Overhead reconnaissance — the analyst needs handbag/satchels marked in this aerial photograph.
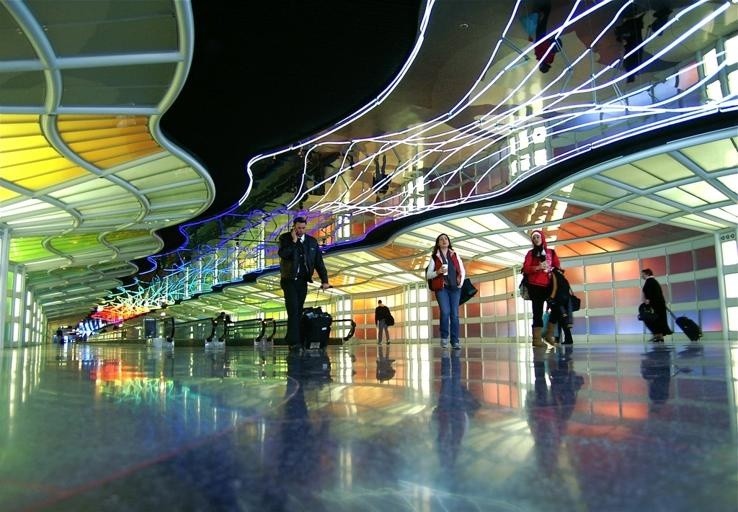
[459,278,478,305]
[570,295,580,312]
[519,273,532,300]
[385,314,394,325]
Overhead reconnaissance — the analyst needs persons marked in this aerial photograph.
[270,351,334,486]
[523,230,561,347]
[432,355,481,487]
[213,311,232,323]
[637,346,672,396]
[613,10,645,83]
[374,300,392,344]
[364,344,397,391]
[551,346,589,447]
[426,234,465,348]
[56,326,63,345]
[553,268,574,344]
[278,216,330,343]
[640,268,666,343]
[371,155,391,204]
[519,351,563,483]
[297,170,310,209]
[526,10,559,75]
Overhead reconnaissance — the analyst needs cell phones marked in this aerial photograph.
[292,225,299,235]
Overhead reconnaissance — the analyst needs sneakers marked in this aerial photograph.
[440,338,463,348]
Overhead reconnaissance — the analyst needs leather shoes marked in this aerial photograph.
[561,340,573,344]
[648,337,664,342]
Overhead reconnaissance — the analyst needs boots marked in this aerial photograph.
[533,326,547,347]
[544,323,554,345]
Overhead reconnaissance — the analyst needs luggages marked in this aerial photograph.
[302,285,333,350]
[666,304,703,341]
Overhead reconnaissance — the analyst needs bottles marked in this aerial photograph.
[442,259,449,277]
[541,250,546,263]
